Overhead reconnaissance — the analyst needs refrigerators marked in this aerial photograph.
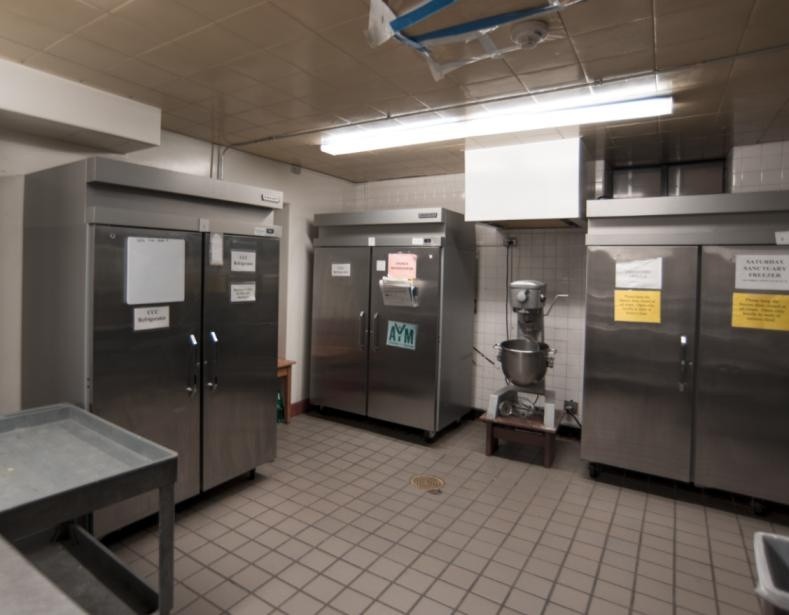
[578,190,788,515]
[22,156,284,542]
[308,206,477,443]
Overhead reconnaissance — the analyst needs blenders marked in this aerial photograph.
[480,280,569,432]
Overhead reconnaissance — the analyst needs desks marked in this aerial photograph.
[277,358,296,424]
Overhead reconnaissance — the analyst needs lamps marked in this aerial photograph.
[319,96,673,156]
